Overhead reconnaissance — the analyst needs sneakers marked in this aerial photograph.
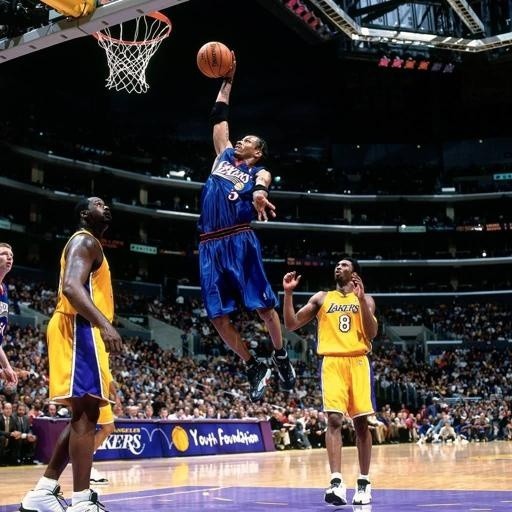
[272,349,297,389]
[245,360,272,402]
[19,490,68,512]
[352,479,371,504]
[325,478,347,505]
[66,492,109,512]
[90,468,109,485]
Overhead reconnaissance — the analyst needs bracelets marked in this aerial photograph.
[222,77,234,84]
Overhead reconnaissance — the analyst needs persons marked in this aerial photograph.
[1,242,19,386]
[109,280,356,451]
[19,193,127,512]
[1,278,59,468]
[196,44,298,403]
[279,252,380,507]
[87,367,123,485]
[367,277,512,446]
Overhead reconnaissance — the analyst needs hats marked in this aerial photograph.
[58,408,68,416]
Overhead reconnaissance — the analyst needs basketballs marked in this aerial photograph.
[197,42,232,79]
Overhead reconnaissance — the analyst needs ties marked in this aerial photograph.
[5,417,9,446]
